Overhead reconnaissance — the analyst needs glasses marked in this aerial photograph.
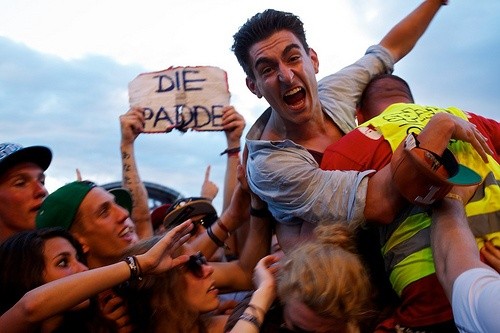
[190,214,216,236]
[178,252,207,278]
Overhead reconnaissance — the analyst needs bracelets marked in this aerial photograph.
[446,193,463,201]
[131,256,142,274]
[125,256,137,278]
[207,227,225,248]
[217,219,233,235]
[247,304,265,315]
[121,259,134,279]
[240,314,260,327]
[220,147,240,156]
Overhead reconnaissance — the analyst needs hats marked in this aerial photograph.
[36,180,96,231]
[162,196,217,229]
[1,142,52,174]
[150,204,171,226]
[389,131,482,205]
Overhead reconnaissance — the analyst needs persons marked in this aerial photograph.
[0,74,500,333]
[231,0,494,231]
